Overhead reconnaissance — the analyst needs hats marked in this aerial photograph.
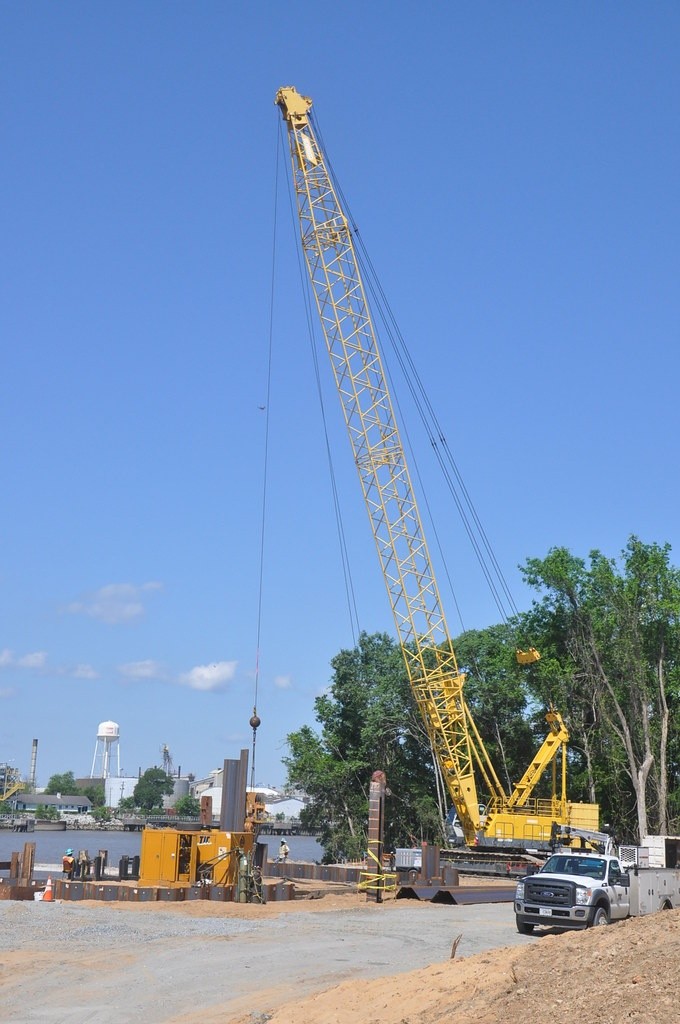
[66,849,73,856]
[281,838,286,842]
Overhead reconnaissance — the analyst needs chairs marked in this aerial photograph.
[62,860,71,880]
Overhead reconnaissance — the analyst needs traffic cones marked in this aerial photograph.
[39,876,57,903]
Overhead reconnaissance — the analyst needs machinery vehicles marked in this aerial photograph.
[274,82,605,879]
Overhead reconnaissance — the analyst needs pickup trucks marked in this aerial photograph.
[513,852,630,934]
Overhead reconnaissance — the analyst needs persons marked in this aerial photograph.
[62,848,76,879]
[75,859,81,878]
[274,838,289,864]
